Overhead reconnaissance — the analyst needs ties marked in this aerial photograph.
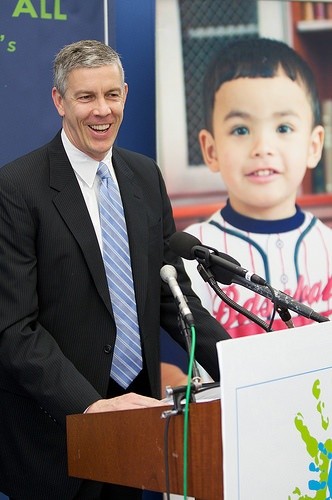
[92,162,146,389]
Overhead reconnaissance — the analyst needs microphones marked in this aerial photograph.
[206,252,330,323]
[169,231,268,288]
[160,265,197,328]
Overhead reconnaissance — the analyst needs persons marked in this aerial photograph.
[173,37,331,337]
[1,37,233,500]
